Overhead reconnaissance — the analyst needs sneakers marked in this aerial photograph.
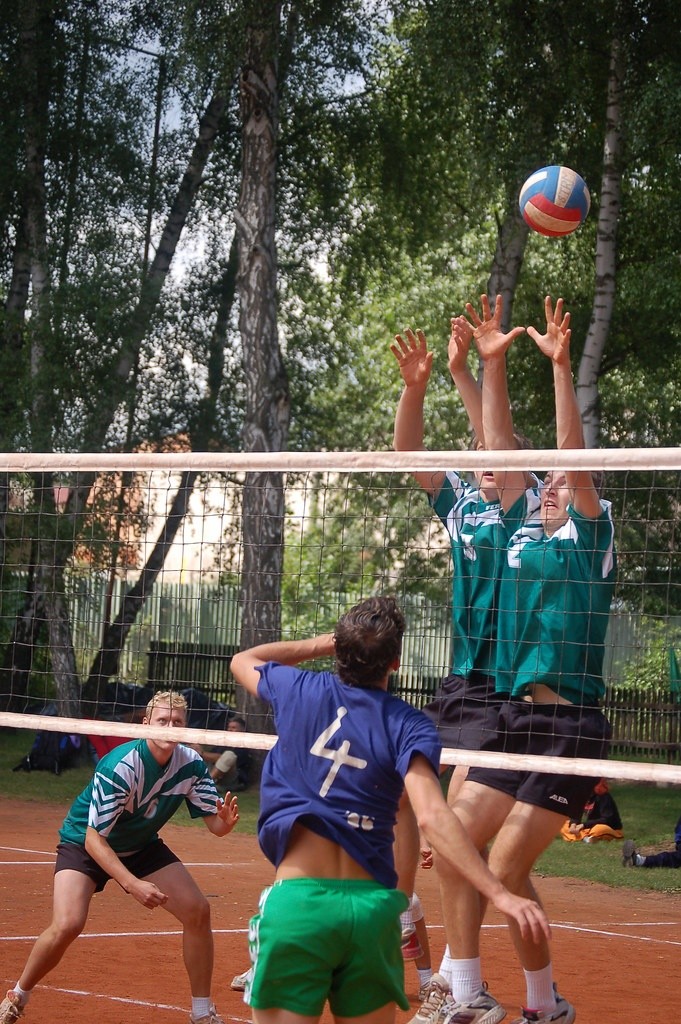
[436,980,507,1024]
[0,990,27,1024]
[230,967,253,991]
[418,979,430,1002]
[189,1002,225,1024]
[621,840,636,867]
[513,982,576,1024]
[409,973,452,1024]
[401,923,424,961]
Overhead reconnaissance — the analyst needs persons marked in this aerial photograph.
[623,817,681,869]
[560,778,624,844]
[186,717,256,791]
[231,597,553,1024]
[231,823,434,1001]
[389,315,543,1024]
[0,691,240,1024]
[432,293,618,1024]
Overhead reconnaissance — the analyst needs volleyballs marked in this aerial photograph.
[518,165,591,237]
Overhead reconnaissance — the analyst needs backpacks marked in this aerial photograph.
[13,728,73,775]
